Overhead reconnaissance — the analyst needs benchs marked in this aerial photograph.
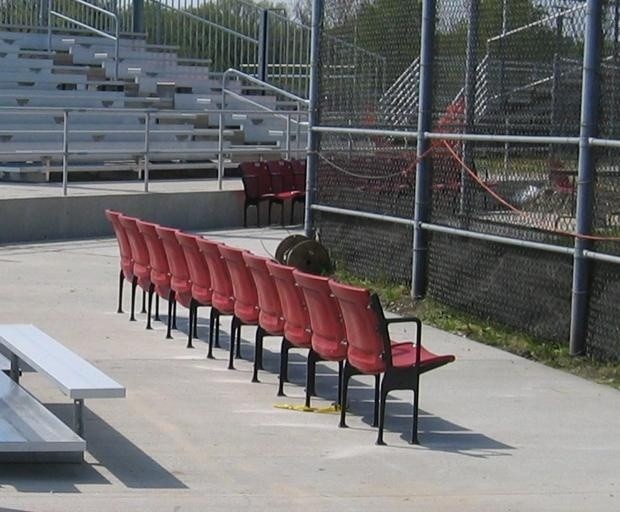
[0,22,385,174]
[0,320,126,440]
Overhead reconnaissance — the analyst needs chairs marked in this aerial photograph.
[175,232,222,349]
[139,220,178,331]
[117,215,161,322]
[330,280,456,446]
[156,226,198,340]
[217,243,264,371]
[292,269,397,409]
[239,159,284,230]
[315,153,499,222]
[197,236,244,358]
[547,156,577,228]
[106,209,146,315]
[290,156,321,226]
[266,158,304,226]
[243,252,289,383]
[267,261,317,398]
